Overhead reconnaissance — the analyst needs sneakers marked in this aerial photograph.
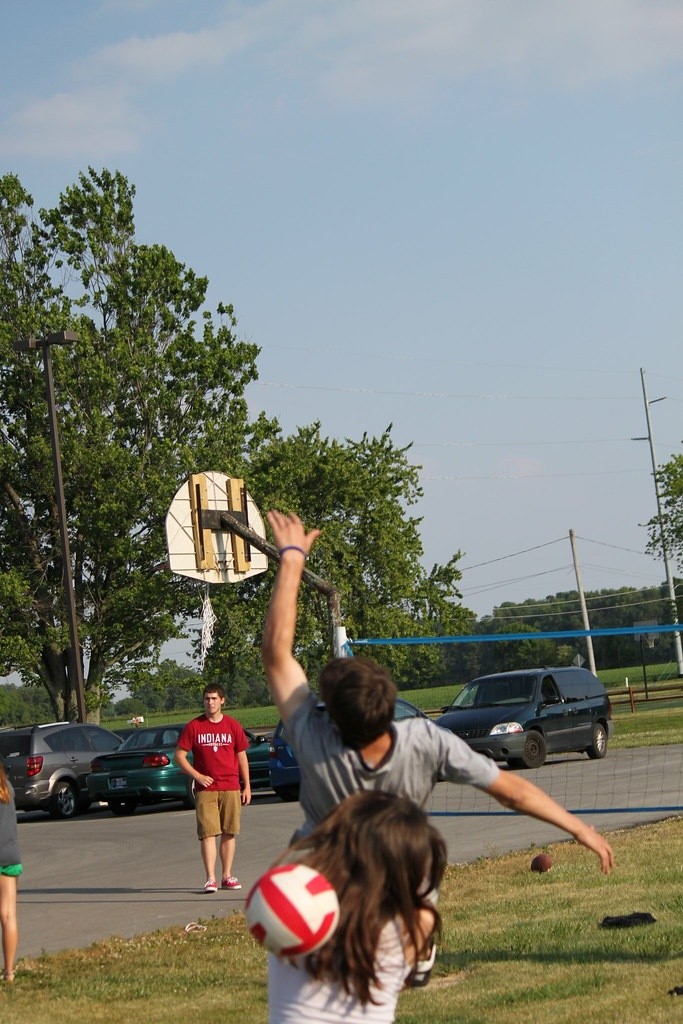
[222,876,242,890]
[410,936,436,986]
[204,878,218,892]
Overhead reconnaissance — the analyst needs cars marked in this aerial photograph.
[112,728,135,740]
[86,723,272,815]
[270,697,434,801]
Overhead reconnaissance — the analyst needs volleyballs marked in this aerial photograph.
[244,864,340,958]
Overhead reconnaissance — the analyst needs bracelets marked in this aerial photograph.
[279,545,306,558]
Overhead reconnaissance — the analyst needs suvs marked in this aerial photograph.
[0,721,130,820]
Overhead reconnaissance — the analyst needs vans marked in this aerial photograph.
[433,667,612,770]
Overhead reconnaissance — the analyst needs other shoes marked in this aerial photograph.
[2,968,15,982]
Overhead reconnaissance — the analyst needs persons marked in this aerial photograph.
[133,716,139,727]
[174,683,251,892]
[268,791,446,1024]
[0,760,22,982]
[261,510,615,987]
